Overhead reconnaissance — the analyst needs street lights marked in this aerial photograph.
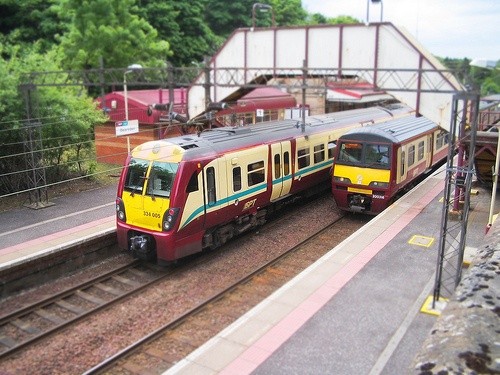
[122,63,142,155]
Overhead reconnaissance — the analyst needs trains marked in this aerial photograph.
[115,100,416,266]
[329,95,499,217]
